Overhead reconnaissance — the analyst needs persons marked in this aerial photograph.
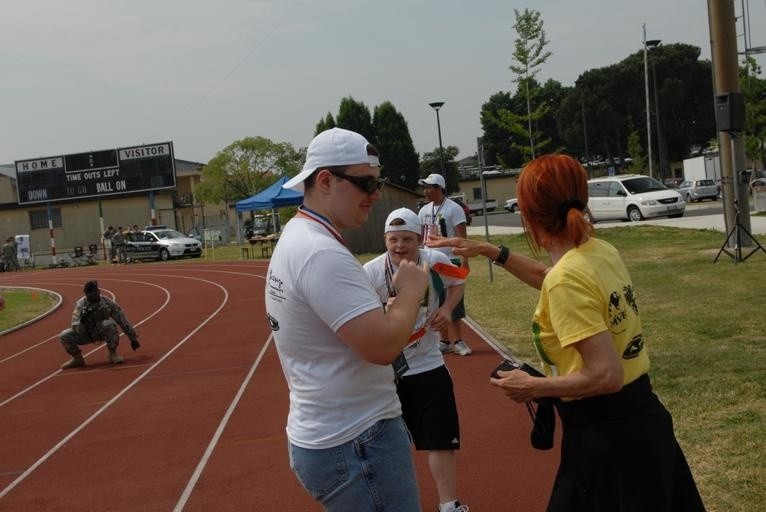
[264,123,430,509]
[362,208,469,511]
[59,279,140,372]
[425,154,705,511]
[415,170,472,356]
[100,222,141,268]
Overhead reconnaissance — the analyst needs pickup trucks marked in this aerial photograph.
[447,193,499,217]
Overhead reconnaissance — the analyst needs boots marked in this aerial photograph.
[60,353,84,369]
[109,348,123,364]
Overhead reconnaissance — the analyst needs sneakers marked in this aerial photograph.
[439,342,452,354]
[454,341,471,355]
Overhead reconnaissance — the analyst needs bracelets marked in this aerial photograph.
[491,244,510,266]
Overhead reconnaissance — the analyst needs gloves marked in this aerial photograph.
[131,340,138,350]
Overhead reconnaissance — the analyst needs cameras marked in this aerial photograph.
[490,359,521,379]
[80,306,93,321]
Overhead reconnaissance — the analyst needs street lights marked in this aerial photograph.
[428,102,447,199]
[642,39,666,184]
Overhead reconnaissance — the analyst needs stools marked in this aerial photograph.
[261,246,268,258]
[241,247,248,259]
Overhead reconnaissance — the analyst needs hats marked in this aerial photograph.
[417,173,445,189]
[280,127,380,192]
[384,208,420,236]
[83,281,96,295]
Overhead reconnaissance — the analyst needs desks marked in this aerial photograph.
[248,235,279,259]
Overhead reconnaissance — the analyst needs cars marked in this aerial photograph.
[582,158,633,168]
[664,176,683,187]
[503,198,521,214]
[251,213,281,242]
[673,177,718,203]
[745,169,765,186]
[104,224,202,261]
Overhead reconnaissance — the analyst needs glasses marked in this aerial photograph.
[331,172,389,194]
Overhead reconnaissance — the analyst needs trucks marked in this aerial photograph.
[682,155,722,195]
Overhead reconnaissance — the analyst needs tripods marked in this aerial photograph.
[713,137,766,265]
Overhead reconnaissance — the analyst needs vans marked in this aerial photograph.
[582,173,686,225]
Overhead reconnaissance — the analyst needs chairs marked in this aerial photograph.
[71,247,83,266]
[85,244,97,265]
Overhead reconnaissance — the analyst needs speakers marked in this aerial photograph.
[715,93,745,131]
